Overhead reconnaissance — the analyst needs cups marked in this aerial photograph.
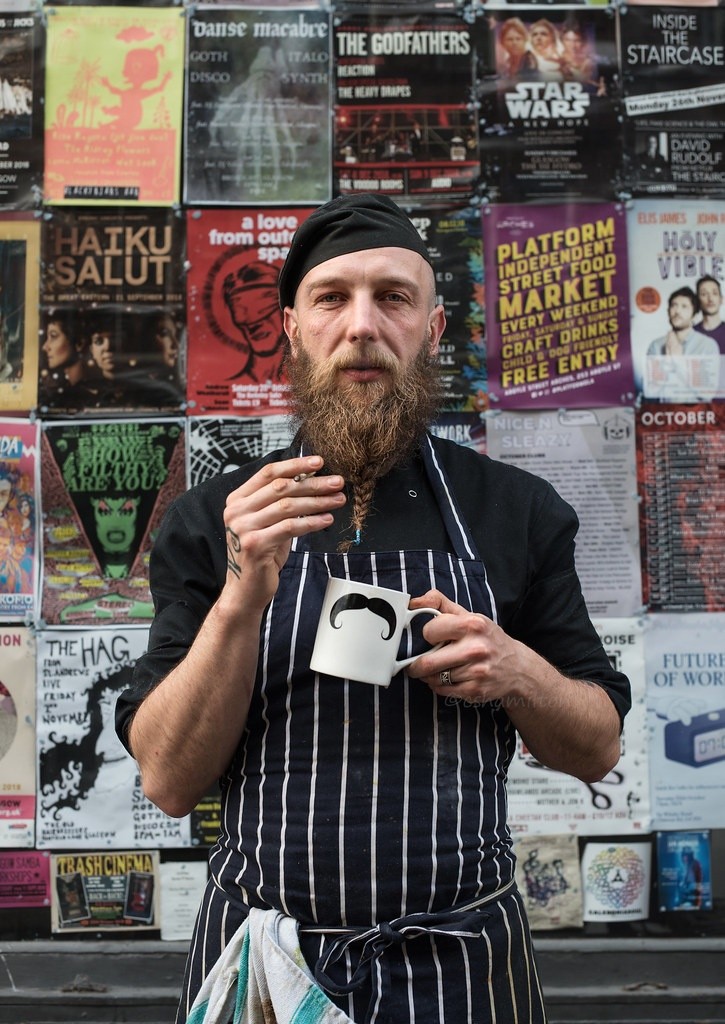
[310,577,446,688]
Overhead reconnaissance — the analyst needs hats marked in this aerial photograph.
[276,193,428,308]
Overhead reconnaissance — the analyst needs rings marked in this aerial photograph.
[439,669,453,685]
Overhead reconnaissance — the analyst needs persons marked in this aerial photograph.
[497,17,615,98]
[648,287,720,403]
[692,273,725,352]
[38,311,185,412]
[119,189,631,1024]
